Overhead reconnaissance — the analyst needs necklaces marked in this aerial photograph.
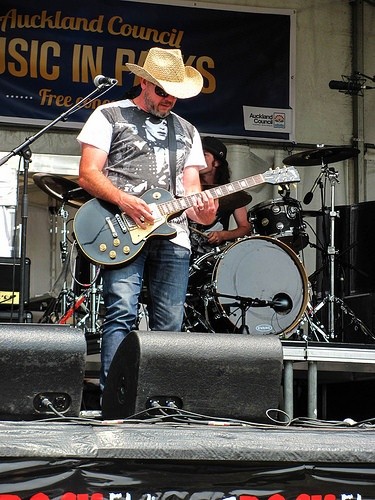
[131,99,137,106]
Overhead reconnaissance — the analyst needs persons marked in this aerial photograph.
[75,47,219,421]
[185,135,253,333]
[143,114,191,195]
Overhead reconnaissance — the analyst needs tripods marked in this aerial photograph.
[310,152,375,342]
[39,188,105,333]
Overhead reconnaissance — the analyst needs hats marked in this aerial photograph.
[201,135,228,161]
[126,47,204,100]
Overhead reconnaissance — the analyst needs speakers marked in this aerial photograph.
[0,256,88,421]
[101,329,283,426]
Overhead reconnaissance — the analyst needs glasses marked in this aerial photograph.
[154,86,170,97]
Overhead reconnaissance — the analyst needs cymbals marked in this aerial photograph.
[32,172,95,209]
[282,147,361,167]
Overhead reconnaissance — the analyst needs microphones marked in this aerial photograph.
[93,74,118,87]
[272,299,289,310]
[329,80,372,90]
[304,172,324,205]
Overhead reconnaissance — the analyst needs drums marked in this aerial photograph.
[246,196,310,253]
[183,235,310,336]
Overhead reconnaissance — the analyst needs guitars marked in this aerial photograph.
[72,165,301,270]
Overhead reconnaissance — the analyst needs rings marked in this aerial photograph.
[140,215,145,222]
[199,207,205,210]
